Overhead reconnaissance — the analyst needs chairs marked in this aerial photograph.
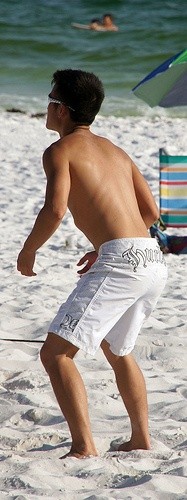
[151,148,187,254]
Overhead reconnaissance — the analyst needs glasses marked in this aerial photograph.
[47,95,76,112]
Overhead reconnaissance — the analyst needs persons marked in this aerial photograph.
[89,18,100,31]
[17,69,168,459]
[73,13,118,32]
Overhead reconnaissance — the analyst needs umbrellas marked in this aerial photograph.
[132,48,187,109]
